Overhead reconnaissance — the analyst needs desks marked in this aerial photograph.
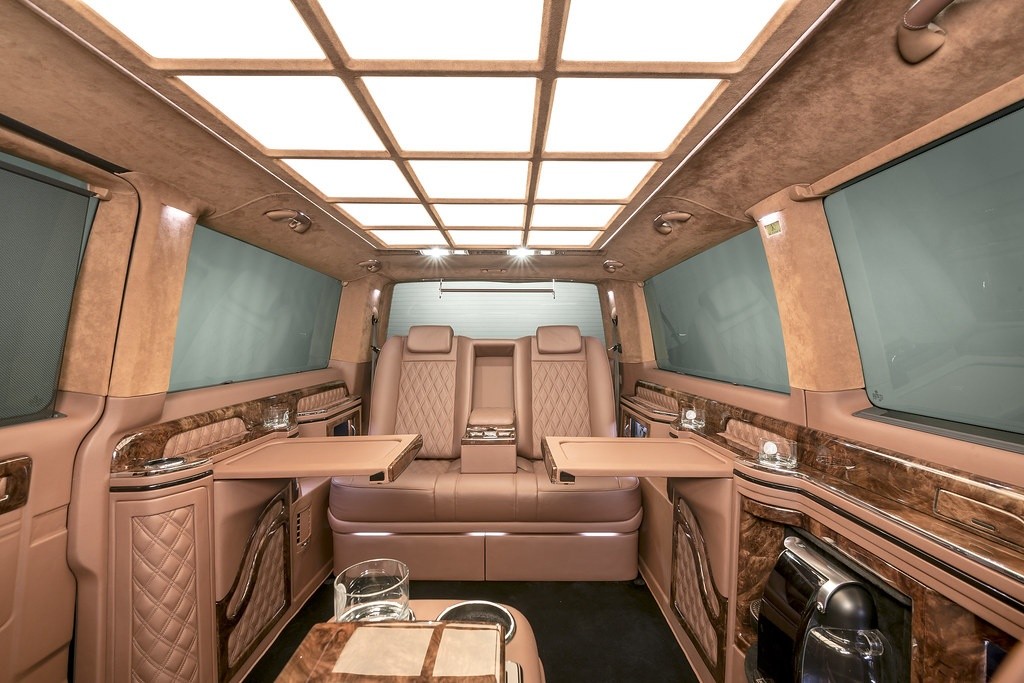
[274,599,548,683]
[542,438,741,683]
[209,434,424,683]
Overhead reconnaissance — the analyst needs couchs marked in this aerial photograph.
[327,326,644,583]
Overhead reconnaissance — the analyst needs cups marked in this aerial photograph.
[758,438,798,470]
[335,557,410,620]
[682,408,706,428]
[264,407,290,431]
